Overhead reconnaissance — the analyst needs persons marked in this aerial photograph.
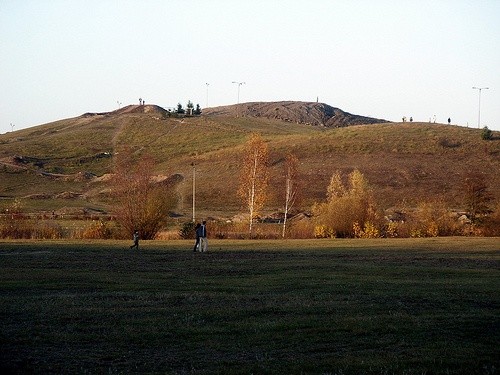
[130,230,139,250]
[193,223,201,252]
[197,221,208,253]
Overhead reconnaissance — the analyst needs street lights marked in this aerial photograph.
[232,81,246,104]
[206,83,210,108]
[472,87,489,129]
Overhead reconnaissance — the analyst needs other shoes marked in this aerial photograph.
[130,246,132,249]
